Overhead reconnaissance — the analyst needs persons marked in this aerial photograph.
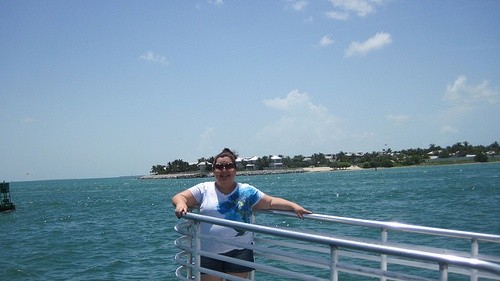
[171,147,312,281]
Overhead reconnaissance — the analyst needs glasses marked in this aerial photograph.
[214,163,234,169]
